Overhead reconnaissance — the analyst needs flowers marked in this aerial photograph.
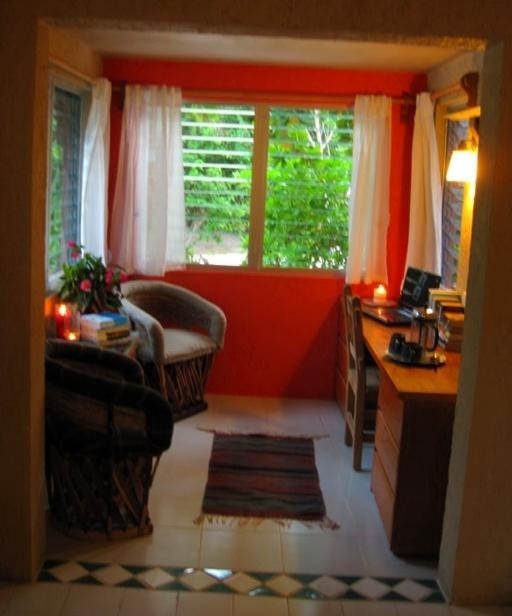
[58,241,127,313]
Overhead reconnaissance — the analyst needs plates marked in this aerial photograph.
[386,349,447,367]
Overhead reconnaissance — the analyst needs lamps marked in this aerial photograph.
[445,139,478,183]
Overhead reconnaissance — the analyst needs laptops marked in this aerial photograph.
[360,268,443,326]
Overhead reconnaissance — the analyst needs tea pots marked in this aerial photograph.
[412,306,439,362]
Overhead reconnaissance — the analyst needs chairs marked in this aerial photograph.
[341,284,382,472]
[45,339,175,543]
[117,280,227,424]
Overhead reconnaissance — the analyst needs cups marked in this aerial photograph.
[401,342,422,363]
[389,333,405,360]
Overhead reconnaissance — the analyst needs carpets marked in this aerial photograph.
[192,427,340,531]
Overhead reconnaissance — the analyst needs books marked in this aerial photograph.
[429,289,464,351]
[79,310,132,348]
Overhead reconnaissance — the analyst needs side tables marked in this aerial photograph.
[83,338,139,357]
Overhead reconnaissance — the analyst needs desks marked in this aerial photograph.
[336,294,461,553]
